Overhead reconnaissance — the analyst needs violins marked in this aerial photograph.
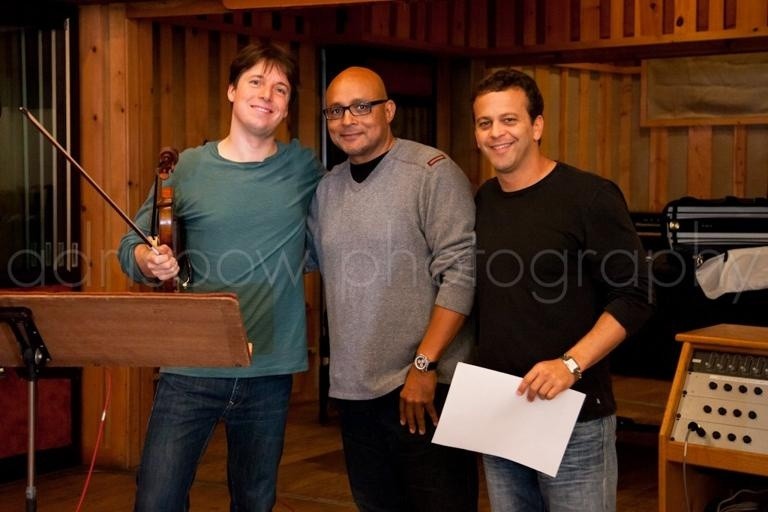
[151,146,178,292]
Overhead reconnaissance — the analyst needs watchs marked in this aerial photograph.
[413,353,438,372]
[559,354,583,381]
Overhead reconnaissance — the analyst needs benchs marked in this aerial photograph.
[611,375,673,432]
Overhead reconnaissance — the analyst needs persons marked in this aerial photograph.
[303,66,480,511]
[467,67,657,512]
[117,37,331,511]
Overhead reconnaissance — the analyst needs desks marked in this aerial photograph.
[657,323,768,512]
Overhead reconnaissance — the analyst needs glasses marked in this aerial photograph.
[323,100,385,120]
